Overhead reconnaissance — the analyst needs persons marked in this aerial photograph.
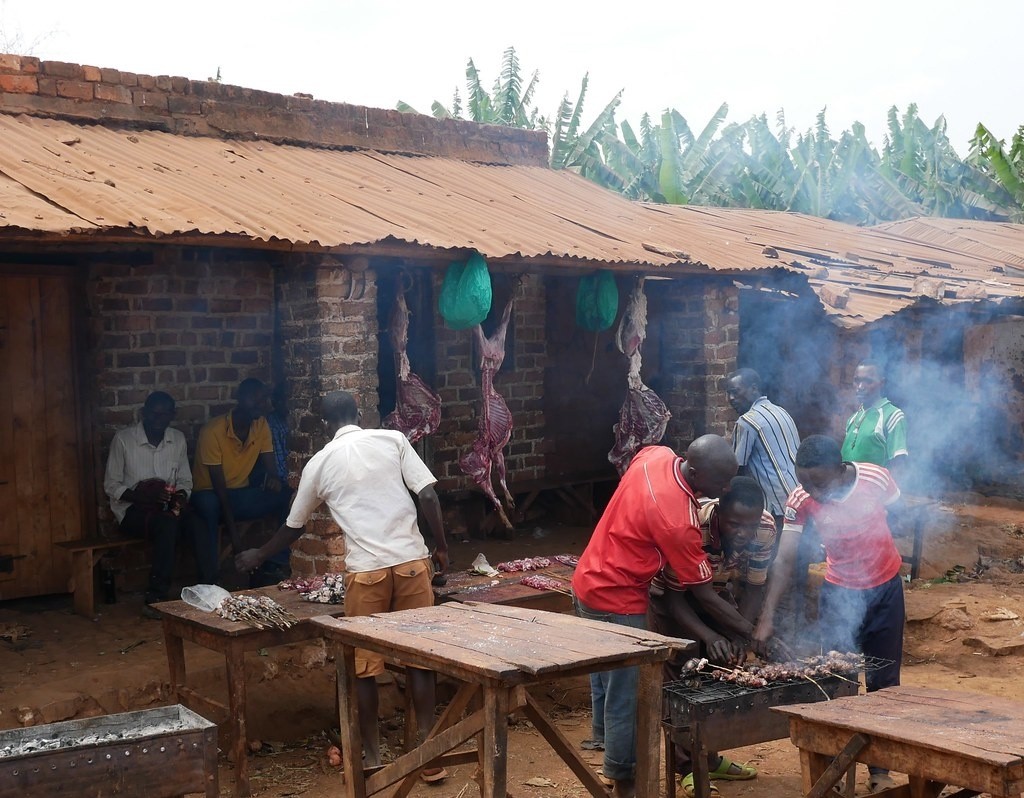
[104,392,193,620]
[725,368,800,583]
[236,392,449,781]
[750,434,955,795]
[194,378,302,592]
[571,434,796,798]
[839,359,909,486]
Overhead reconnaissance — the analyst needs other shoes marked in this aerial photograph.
[144,591,169,616]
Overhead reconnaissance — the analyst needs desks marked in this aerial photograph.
[768,685,1024,798]
[810,492,938,583]
[147,551,698,798]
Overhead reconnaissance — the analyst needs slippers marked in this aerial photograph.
[679,771,720,798]
[864,773,899,794]
[420,766,447,782]
[707,755,757,779]
[832,779,846,797]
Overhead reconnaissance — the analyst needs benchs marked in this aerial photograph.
[53,518,275,620]
[471,468,619,540]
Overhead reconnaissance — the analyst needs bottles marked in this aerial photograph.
[160,467,179,510]
